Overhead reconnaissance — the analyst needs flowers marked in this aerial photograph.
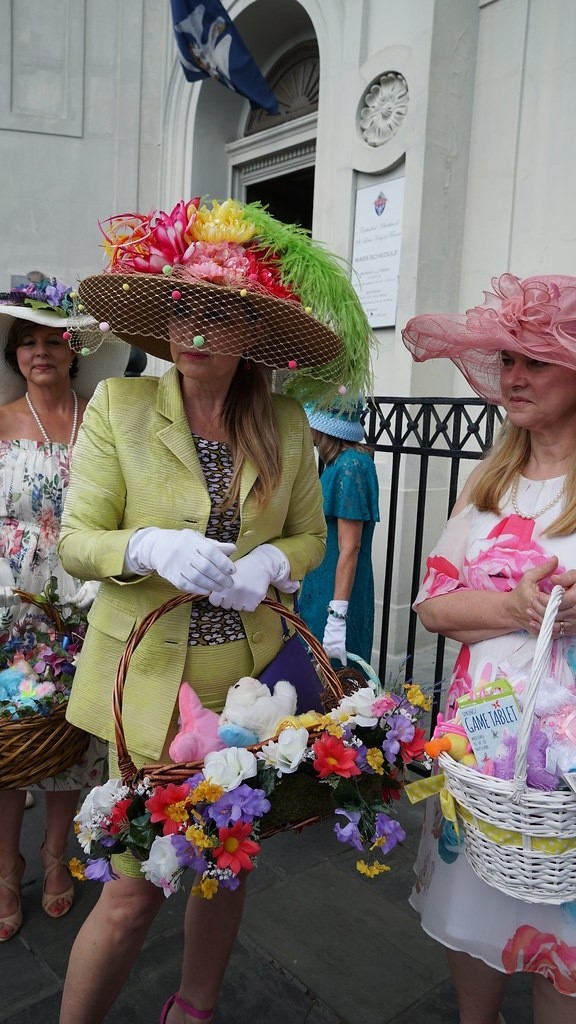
[69,654,452,899]
[0,577,88,724]
[91,195,305,306]
[0,272,80,319]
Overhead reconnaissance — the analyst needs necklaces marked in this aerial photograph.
[512,463,567,520]
[26,389,78,447]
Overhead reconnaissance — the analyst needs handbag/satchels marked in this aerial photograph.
[430,581,576,906]
[112,591,347,870]
[0,589,91,792]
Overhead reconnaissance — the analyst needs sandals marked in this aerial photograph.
[38,829,75,918]
[0,851,27,942]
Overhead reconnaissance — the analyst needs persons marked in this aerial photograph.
[296,386,378,670]
[55,208,366,1024]
[0,278,106,941]
[400,274,576,1024]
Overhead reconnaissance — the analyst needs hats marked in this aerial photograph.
[406,276,576,406]
[0,272,132,408]
[302,391,365,441]
[63,194,379,419]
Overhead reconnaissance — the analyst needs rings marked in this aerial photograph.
[560,631,564,636]
[559,622,565,630]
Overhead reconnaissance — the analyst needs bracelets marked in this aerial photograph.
[326,605,348,619]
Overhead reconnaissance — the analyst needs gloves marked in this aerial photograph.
[0,558,22,606]
[207,543,301,612]
[126,526,237,593]
[66,580,101,611]
[323,600,349,666]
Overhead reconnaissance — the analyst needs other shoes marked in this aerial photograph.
[160,992,215,1024]
